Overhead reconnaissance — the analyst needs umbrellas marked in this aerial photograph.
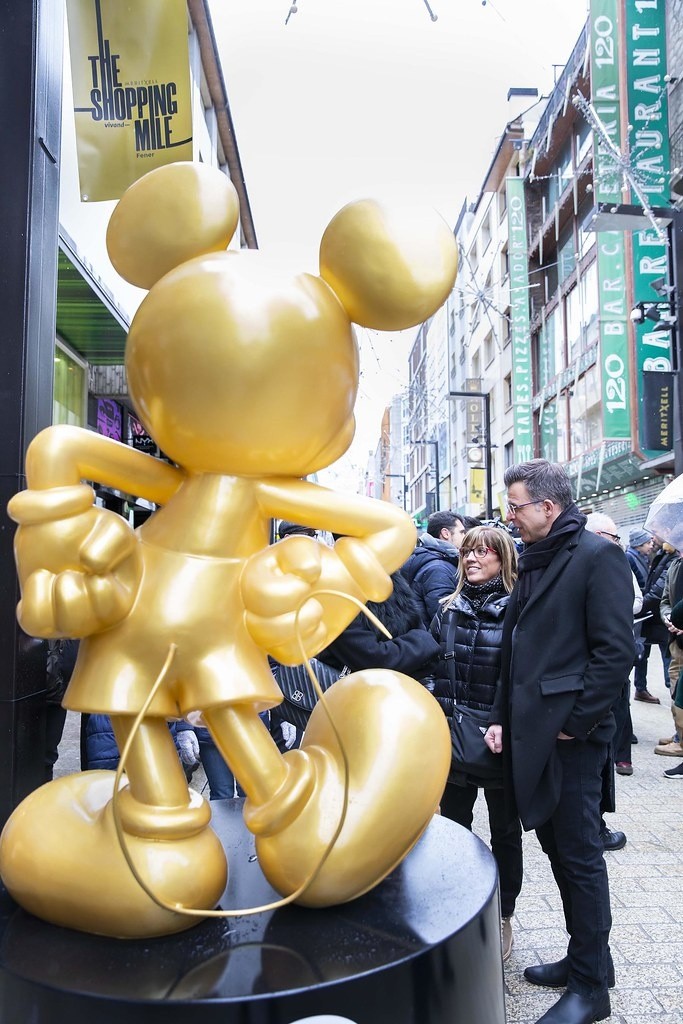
[643,473,683,553]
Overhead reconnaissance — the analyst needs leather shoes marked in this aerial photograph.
[600,828,627,851]
[523,947,615,989]
[634,689,660,704]
[535,984,611,1024]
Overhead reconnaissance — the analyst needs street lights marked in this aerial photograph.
[385,474,408,511]
[409,440,441,512]
[445,390,492,520]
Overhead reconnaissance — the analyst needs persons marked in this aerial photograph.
[44,461,683,1024]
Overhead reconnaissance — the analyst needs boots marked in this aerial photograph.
[658,701,683,744]
[654,704,683,757]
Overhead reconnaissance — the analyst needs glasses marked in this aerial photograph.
[506,498,556,515]
[291,530,315,538]
[601,530,621,544]
[459,545,499,558]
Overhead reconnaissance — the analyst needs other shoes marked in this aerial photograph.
[500,913,515,962]
[616,759,633,775]
[630,734,638,744]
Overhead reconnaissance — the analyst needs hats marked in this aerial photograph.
[629,527,653,549]
[278,521,317,539]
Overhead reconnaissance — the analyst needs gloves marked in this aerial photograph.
[175,731,199,766]
[280,721,297,750]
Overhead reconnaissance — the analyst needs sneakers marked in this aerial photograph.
[663,762,683,779]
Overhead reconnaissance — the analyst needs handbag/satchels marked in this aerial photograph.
[450,704,512,784]
[269,653,348,730]
